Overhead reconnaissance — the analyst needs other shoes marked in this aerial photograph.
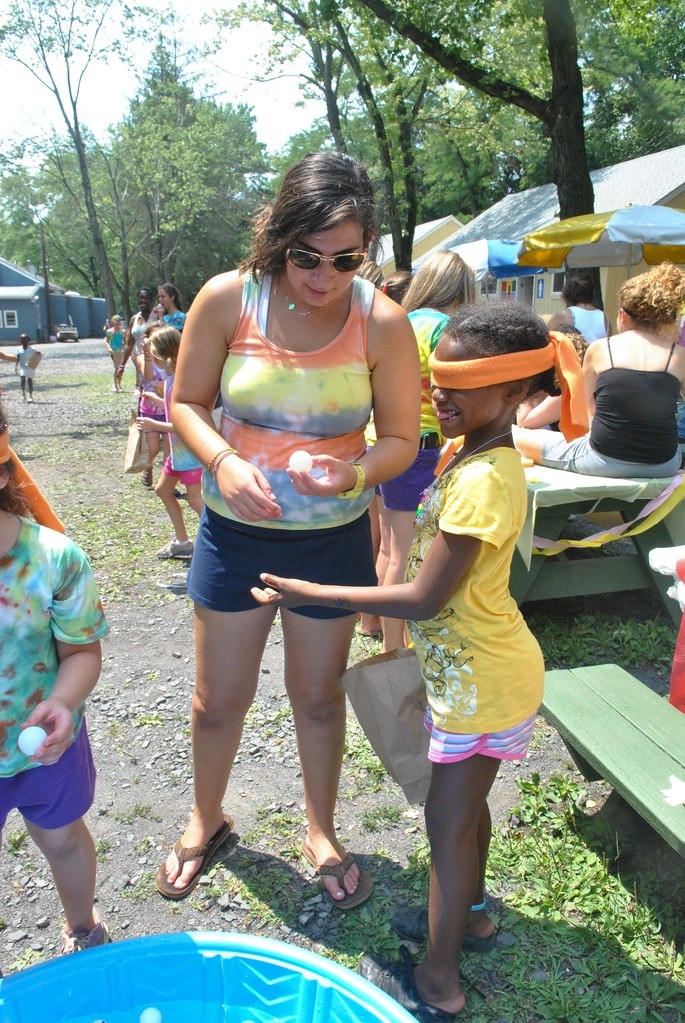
[21,391,26,400]
[28,395,34,402]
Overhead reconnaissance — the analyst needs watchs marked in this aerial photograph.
[336,463,365,499]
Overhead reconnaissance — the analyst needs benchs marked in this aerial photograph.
[537,664,685,861]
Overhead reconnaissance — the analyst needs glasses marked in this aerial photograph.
[286,248,366,273]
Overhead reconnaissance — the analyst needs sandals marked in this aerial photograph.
[141,466,153,486]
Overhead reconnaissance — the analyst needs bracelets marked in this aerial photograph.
[118,365,125,367]
[209,449,239,479]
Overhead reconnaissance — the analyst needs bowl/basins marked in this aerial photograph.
[0,930,421,1023]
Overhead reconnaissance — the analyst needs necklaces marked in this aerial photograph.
[440,431,512,478]
[284,286,326,316]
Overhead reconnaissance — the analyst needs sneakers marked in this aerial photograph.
[394,905,499,951]
[358,945,457,1023]
[157,538,195,560]
[60,926,110,953]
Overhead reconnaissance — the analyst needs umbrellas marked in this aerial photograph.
[518,202,685,279]
[450,236,546,299]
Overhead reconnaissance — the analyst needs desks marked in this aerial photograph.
[508,460,685,631]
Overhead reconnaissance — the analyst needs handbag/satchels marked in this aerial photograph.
[340,649,434,805]
[123,421,152,473]
[113,351,124,368]
[25,351,43,370]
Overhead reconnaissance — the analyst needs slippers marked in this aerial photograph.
[300,834,375,911]
[157,811,234,899]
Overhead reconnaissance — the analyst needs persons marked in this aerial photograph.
[361,250,474,658]
[116,284,204,560]
[105,315,127,392]
[548,271,613,343]
[512,259,685,478]
[249,303,591,1023]
[0,406,109,950]
[158,150,421,912]
[15,334,40,402]
[0,352,17,363]
